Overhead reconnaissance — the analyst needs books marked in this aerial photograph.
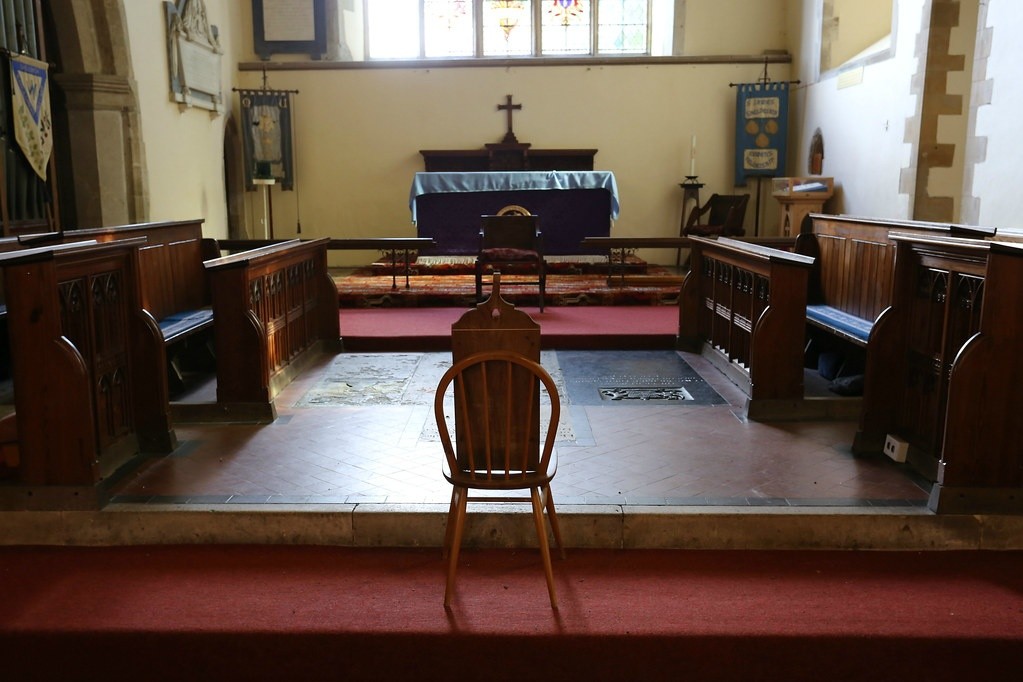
[784,182,827,192]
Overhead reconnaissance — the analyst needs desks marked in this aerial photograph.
[771,176,835,252]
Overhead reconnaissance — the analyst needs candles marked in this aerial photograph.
[690,132,697,176]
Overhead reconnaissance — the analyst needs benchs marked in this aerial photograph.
[806,296,875,354]
[160,303,214,354]
[214,235,439,290]
[581,235,800,287]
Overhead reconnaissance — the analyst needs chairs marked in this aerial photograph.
[683,192,750,238]
[473,214,548,315]
[440,350,571,614]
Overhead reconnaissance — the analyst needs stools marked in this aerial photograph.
[676,183,706,268]
